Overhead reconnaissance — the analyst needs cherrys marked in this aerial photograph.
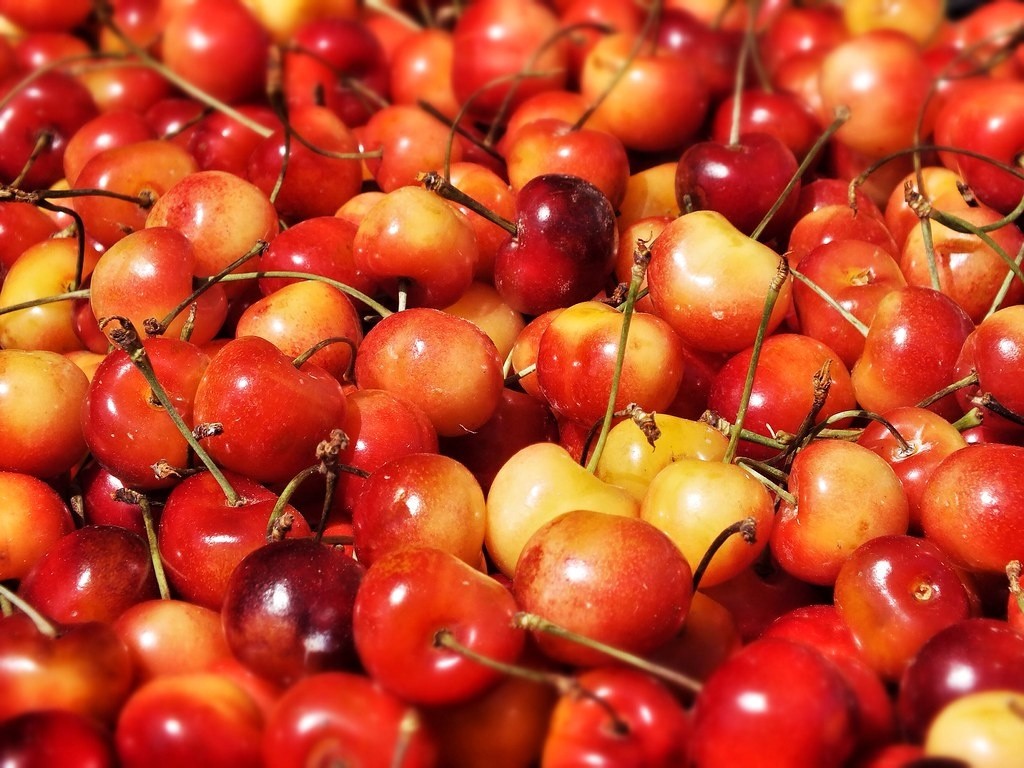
[1,1,1024,768]
